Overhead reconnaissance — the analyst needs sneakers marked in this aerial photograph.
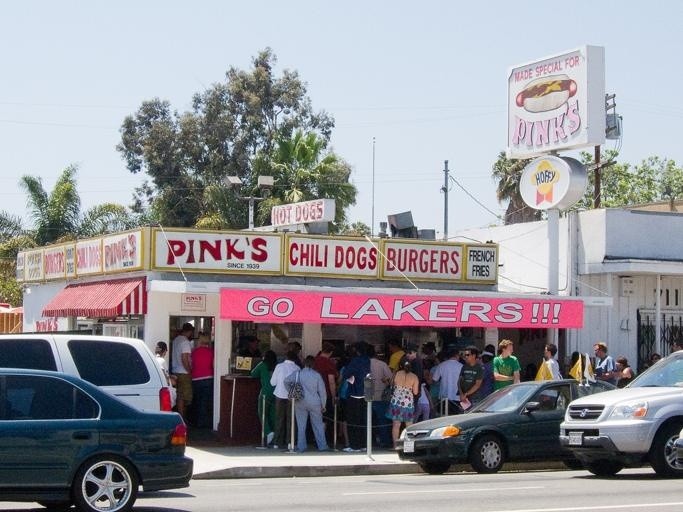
[265,432,274,445]
[359,448,366,452]
[342,446,360,452]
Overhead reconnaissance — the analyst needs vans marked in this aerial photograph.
[0,334,177,414]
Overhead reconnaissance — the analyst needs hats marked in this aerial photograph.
[182,322,195,330]
[154,341,166,355]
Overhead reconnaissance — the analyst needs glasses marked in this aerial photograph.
[464,354,471,357]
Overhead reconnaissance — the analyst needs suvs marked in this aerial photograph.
[560,350,683,479]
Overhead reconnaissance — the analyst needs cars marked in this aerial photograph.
[395,379,616,474]
[0,368,192,511]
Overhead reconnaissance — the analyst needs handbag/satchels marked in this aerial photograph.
[166,386,177,408]
[288,384,304,401]
[381,384,394,406]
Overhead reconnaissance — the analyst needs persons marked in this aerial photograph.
[673,339,683,352]
[639,353,671,387]
[154,324,638,452]
